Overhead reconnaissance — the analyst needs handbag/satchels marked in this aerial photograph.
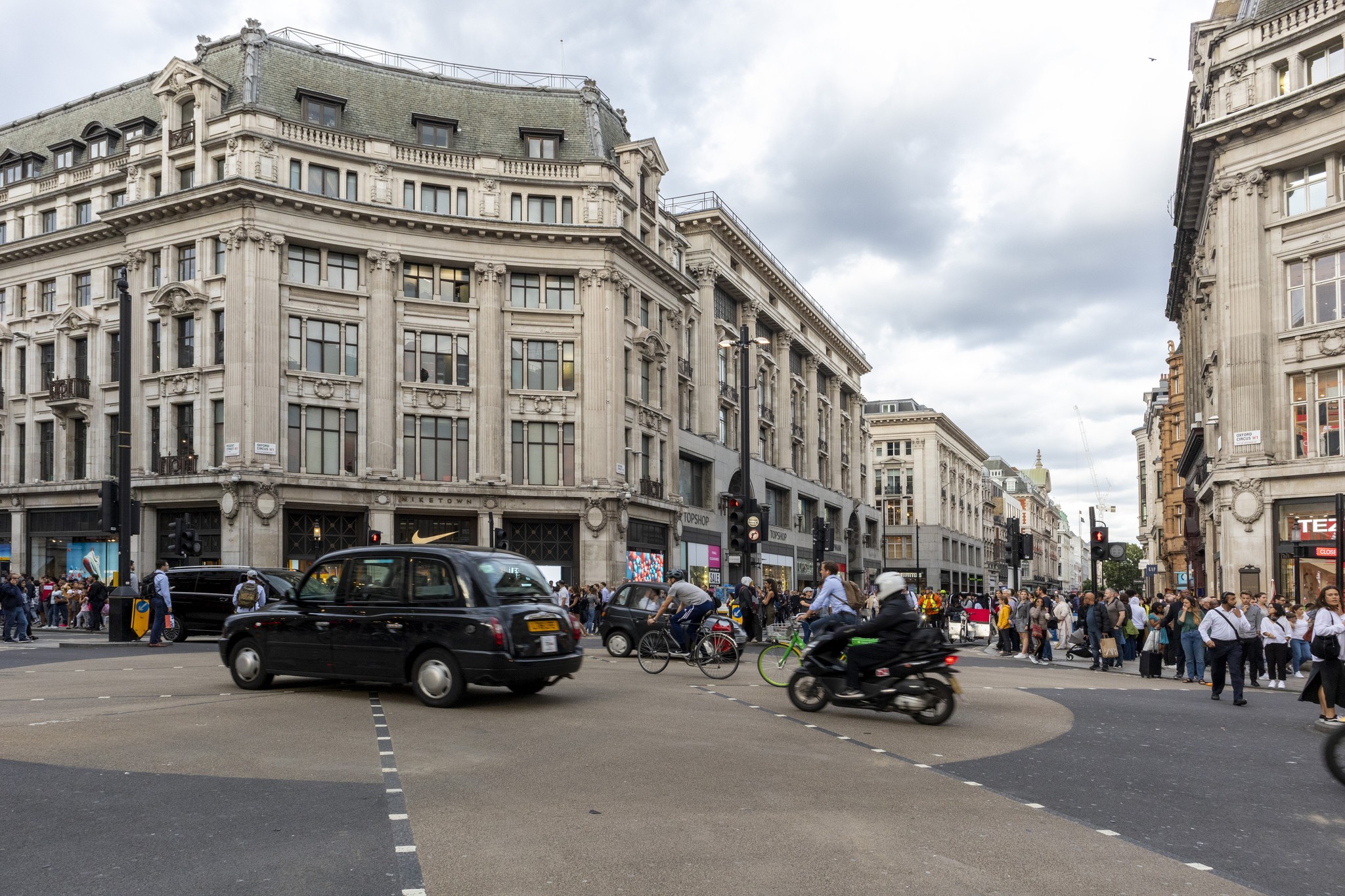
[1032,615,1043,638]
[165,612,175,628]
[54,595,68,605]
[1100,635,1118,658]
[1310,609,1341,661]
[1143,630,1160,650]
[753,602,757,610]
[1047,616,1059,629]
[1303,625,1314,641]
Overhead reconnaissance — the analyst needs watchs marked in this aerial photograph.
[1115,625,1119,627]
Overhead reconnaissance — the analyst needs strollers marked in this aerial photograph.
[1066,628,1102,661]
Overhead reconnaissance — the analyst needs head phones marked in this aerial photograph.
[1323,602,1335,610]
[1221,592,1228,604]
[1186,595,1195,607]
[1268,614,1278,620]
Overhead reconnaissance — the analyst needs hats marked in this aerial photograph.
[246,570,258,578]
[803,587,814,593]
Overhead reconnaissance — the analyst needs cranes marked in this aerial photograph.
[1073,404,1116,527]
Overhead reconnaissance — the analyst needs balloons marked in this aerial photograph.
[626,551,663,582]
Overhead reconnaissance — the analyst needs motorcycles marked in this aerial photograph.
[786,623,963,726]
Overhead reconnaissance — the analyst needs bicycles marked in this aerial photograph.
[637,610,740,680]
[757,613,879,688]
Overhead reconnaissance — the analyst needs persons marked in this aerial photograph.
[1120,588,1345,688]
[647,568,714,656]
[990,579,1126,671]
[796,561,858,637]
[549,577,717,638]
[315,571,453,606]
[233,570,266,615]
[834,571,920,697]
[726,577,994,647]
[0,573,109,643]
[130,560,138,593]
[1198,591,1251,706]
[1298,586,1345,726]
[149,560,173,647]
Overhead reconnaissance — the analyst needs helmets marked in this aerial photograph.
[940,590,946,594]
[665,569,684,578]
[874,572,907,600]
[925,586,933,590]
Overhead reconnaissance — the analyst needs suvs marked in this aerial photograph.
[219,543,586,709]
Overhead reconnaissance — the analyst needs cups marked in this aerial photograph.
[1045,615,1050,619]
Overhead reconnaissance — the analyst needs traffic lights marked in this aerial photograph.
[730,495,747,552]
[1005,518,1012,542]
[97,481,119,533]
[494,528,507,550]
[1090,527,1108,561]
[1005,540,1018,566]
[367,530,381,546]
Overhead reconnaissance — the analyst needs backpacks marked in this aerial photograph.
[1116,600,1128,627]
[580,593,588,610]
[713,596,722,608]
[96,582,108,601]
[237,582,259,607]
[830,577,865,610]
[141,571,164,598]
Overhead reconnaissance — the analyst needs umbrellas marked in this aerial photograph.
[1270,578,1276,603]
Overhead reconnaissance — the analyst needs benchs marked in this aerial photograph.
[948,608,990,624]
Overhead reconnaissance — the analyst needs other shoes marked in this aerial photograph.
[0,619,167,647]
[579,632,1345,728]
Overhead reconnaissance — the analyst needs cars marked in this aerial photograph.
[598,582,748,661]
[143,565,332,643]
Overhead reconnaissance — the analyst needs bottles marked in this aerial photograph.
[726,598,730,603]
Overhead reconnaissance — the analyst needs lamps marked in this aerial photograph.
[232,474,241,482]
[844,528,854,544]
[760,503,772,512]
[379,475,387,479]
[794,514,804,533]
[718,492,734,516]
[488,481,495,484]
[1205,415,1218,425]
[824,521,830,527]
[881,538,887,548]
[863,533,871,548]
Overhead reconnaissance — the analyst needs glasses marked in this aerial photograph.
[954,603,960,605]
[1267,607,1273,610]
[807,591,813,593]
[874,591,878,593]
[941,593,945,595]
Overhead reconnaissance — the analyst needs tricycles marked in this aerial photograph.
[947,592,992,646]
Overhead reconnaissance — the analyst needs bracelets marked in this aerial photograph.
[1268,633,1271,636]
[762,600,764,602]
[653,617,656,622]
[597,597,599,598]
[1160,618,1162,620]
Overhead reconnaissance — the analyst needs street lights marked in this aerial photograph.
[1290,517,1303,604]
[717,324,771,642]
[882,496,912,572]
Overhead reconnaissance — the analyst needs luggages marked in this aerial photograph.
[1139,628,1162,678]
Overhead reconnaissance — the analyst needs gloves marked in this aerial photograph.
[934,607,937,610]
